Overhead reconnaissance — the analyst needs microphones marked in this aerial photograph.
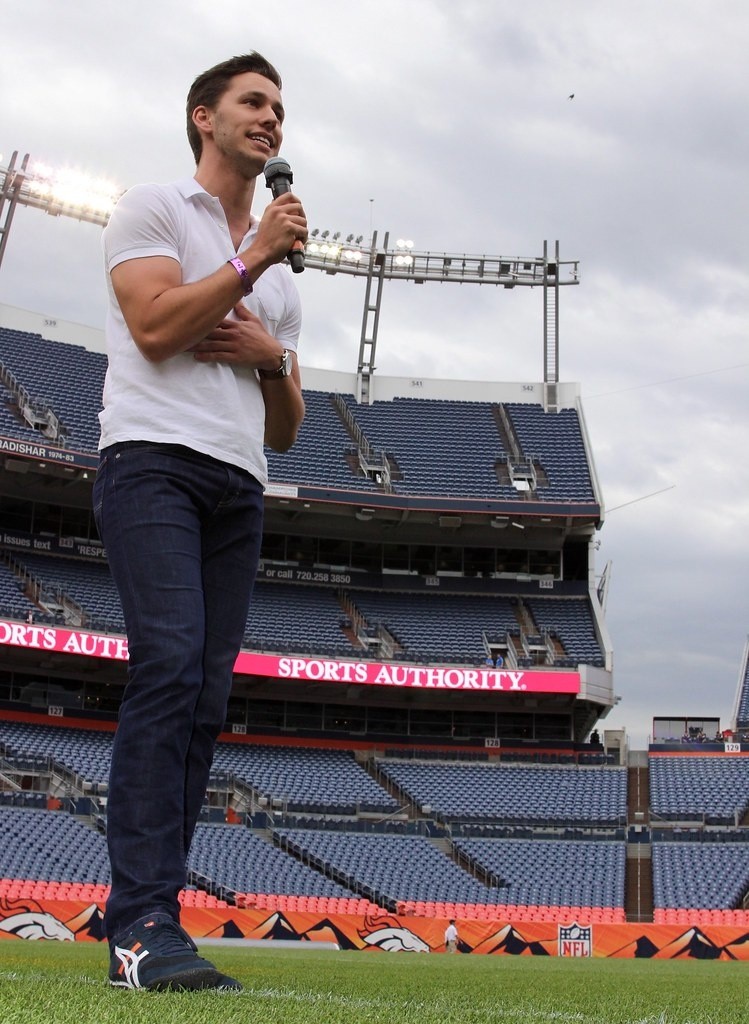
[264,156,305,273]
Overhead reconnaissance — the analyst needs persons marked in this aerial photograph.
[90,54,306,990]
[715,731,723,743]
[590,729,599,743]
[444,919,459,953]
[486,654,494,669]
[496,654,503,669]
[696,731,707,743]
[25,610,35,624]
[682,731,692,743]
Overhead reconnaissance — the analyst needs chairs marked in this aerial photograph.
[0,327,749,929]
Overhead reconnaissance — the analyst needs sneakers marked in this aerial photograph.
[207,971,243,992]
[108,912,217,992]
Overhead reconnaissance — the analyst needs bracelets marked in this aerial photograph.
[227,257,253,297]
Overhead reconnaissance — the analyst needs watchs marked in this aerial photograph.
[258,349,293,380]
